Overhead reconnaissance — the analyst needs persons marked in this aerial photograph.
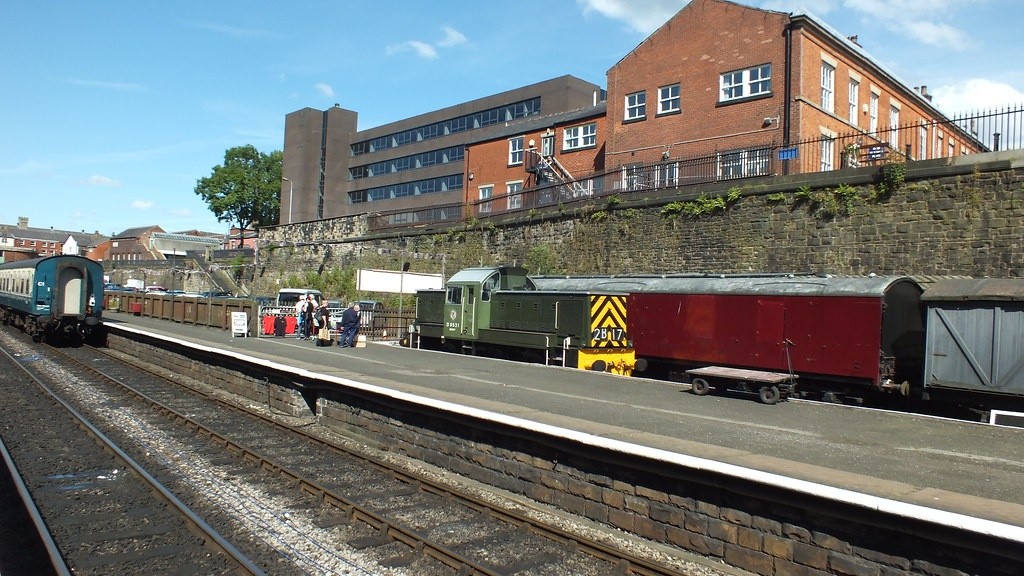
[316,299,329,343]
[301,296,314,341]
[295,295,309,340]
[307,294,320,339]
[338,301,358,348]
[344,303,361,347]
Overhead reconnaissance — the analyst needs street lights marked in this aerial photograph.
[282,177,293,224]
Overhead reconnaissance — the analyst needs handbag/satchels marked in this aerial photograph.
[318,326,330,340]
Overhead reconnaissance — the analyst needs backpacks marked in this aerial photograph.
[315,313,324,325]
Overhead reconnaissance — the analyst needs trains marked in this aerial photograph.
[0,254,104,344]
[401,266,1024,431]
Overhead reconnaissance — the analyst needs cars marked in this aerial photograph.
[103,283,386,330]
